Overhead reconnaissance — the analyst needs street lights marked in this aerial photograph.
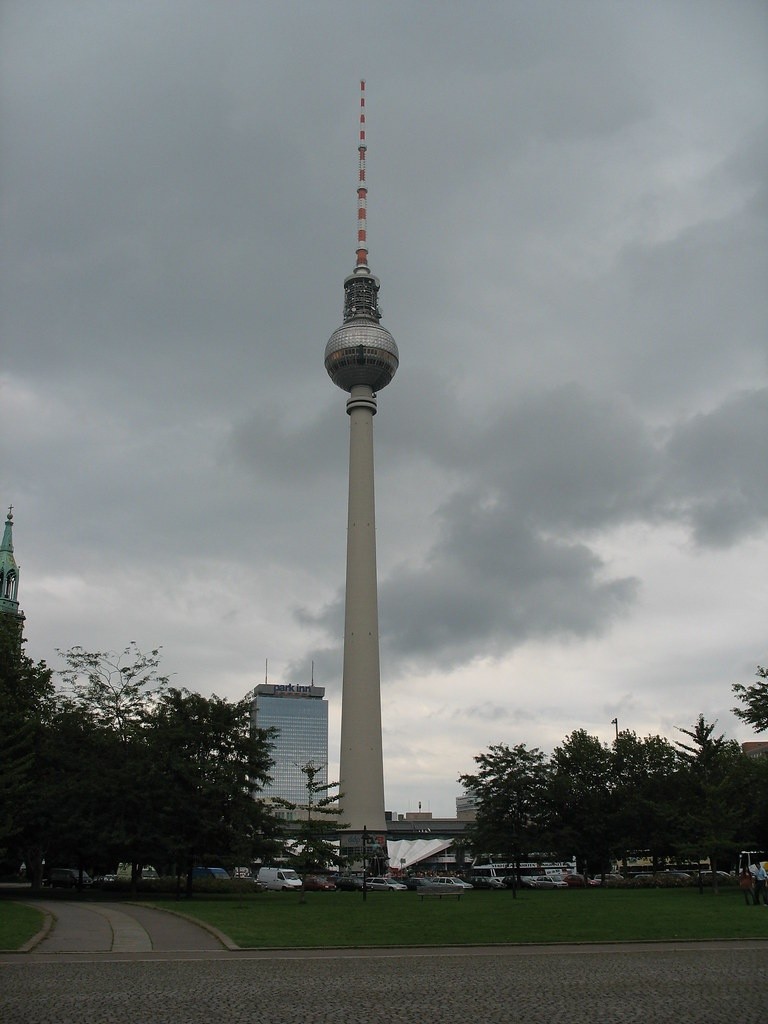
[611,717,619,741]
[361,824,370,900]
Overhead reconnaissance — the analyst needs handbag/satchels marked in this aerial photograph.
[739,875,752,889]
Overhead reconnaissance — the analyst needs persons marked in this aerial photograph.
[752,861,768,906]
[740,865,757,905]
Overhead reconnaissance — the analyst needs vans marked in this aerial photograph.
[47,868,94,889]
[698,870,731,878]
[193,867,231,881]
[116,862,160,883]
[258,867,303,892]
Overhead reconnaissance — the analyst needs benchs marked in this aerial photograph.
[415,885,463,902]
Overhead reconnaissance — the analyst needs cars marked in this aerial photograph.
[563,874,601,888]
[594,874,624,883]
[366,876,409,892]
[405,877,430,891]
[534,875,569,889]
[666,873,692,883]
[102,874,118,882]
[234,876,268,890]
[301,877,338,891]
[470,875,537,889]
[335,877,366,890]
[429,877,475,890]
